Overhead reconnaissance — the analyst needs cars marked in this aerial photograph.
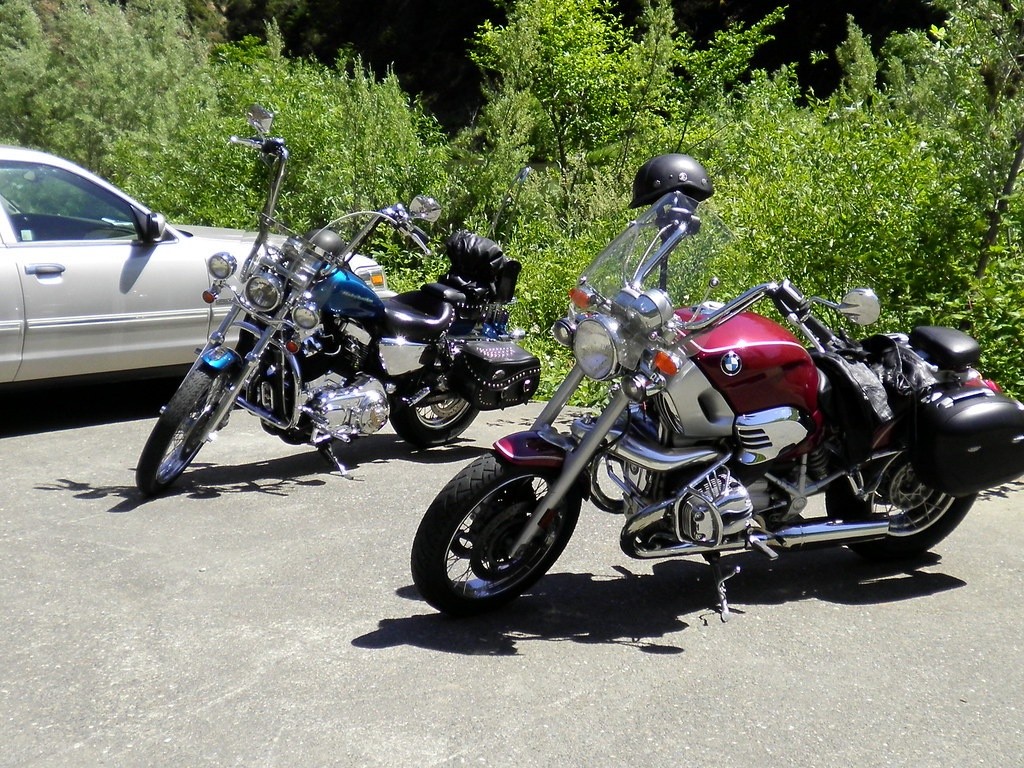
[0,148,398,384]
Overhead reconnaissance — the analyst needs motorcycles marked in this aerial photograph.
[410,189,1024,623]
[134,103,542,498]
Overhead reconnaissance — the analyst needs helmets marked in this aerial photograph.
[306,229,345,255]
[627,153,714,209]
